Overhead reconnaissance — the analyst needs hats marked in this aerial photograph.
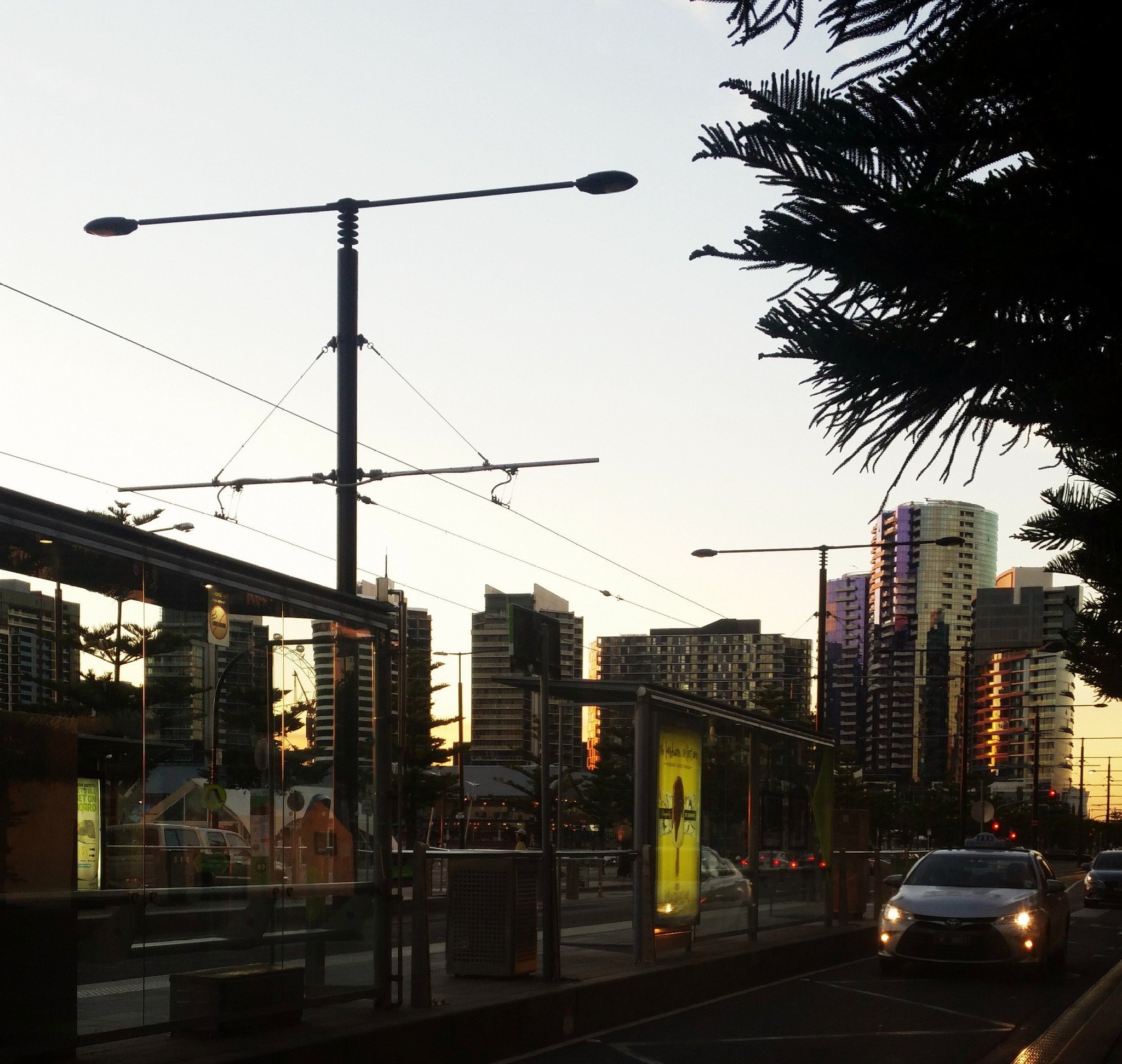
[515,829,528,836]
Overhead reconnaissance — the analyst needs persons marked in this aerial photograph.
[514,828,526,850]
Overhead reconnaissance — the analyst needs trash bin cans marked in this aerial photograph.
[446,856,539,979]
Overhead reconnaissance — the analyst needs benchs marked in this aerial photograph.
[129,926,363,986]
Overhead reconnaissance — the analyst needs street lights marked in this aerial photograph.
[969,704,1109,851]
[83,170,641,932]
[692,536,969,783]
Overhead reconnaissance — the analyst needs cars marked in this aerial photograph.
[1080,850,1122,908]
[877,833,1070,981]
[799,856,817,866]
[740,850,797,870]
[699,846,752,911]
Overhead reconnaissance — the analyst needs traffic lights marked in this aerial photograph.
[1049,790,1056,806]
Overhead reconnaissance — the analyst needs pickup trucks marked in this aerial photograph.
[101,823,290,891]
